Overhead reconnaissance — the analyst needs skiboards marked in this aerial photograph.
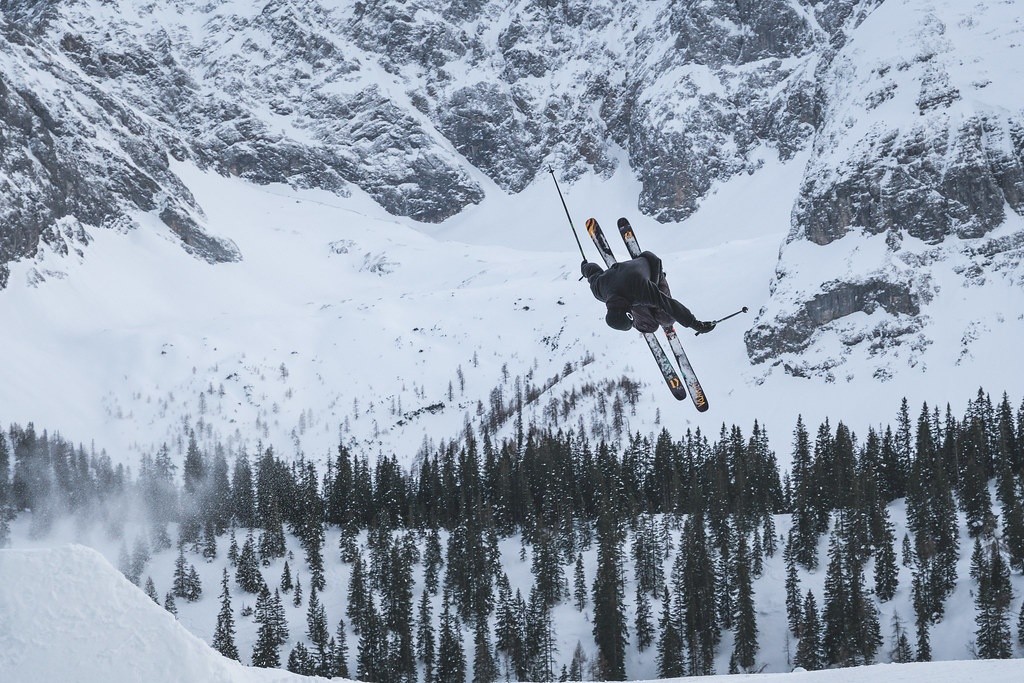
[584,215,711,415]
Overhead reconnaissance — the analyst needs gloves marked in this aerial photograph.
[690,318,716,333]
[581,260,588,272]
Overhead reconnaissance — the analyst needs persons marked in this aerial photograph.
[581,251,716,334]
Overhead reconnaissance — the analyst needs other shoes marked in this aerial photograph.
[664,326,677,340]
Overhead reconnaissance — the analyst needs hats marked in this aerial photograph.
[606,304,634,331]
